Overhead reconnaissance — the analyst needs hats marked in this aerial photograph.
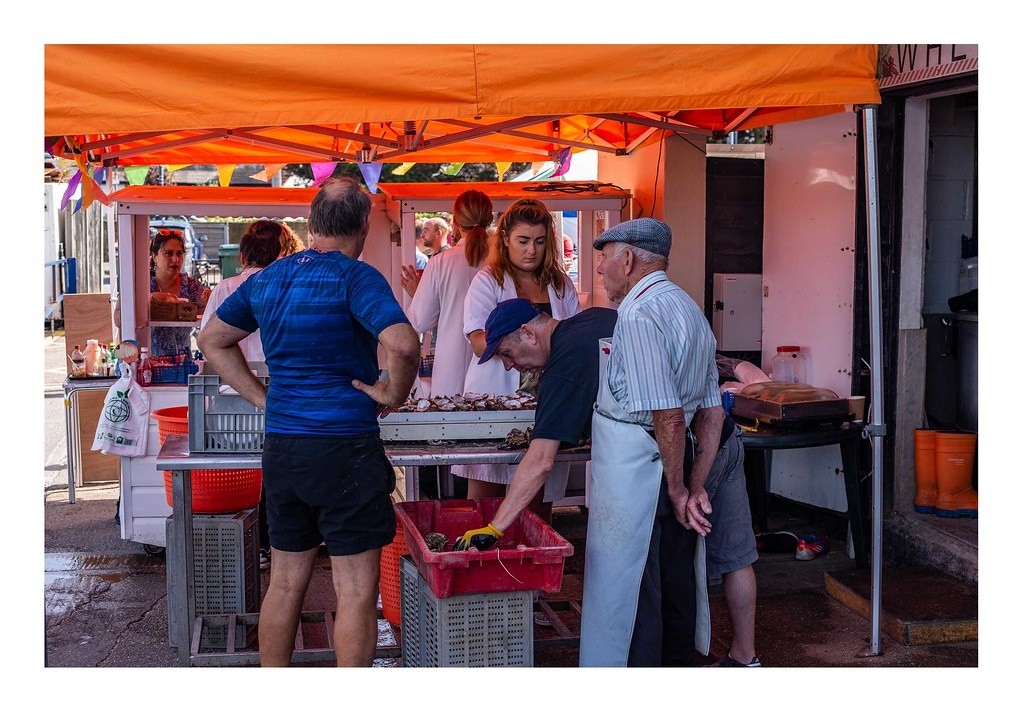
[592,217,672,257]
[477,297,544,365]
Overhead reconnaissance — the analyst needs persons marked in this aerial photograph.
[201,221,283,361]
[115,230,212,524]
[197,177,421,667]
[579,218,725,667]
[452,298,762,667]
[408,212,452,397]
[452,198,581,532]
[400,189,498,398]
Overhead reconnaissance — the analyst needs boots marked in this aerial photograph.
[935,431,977,519]
[913,429,944,514]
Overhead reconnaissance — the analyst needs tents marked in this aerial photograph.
[45,44,885,658]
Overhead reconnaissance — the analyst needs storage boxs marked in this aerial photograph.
[150,300,198,321]
[734,394,850,418]
[188,360,271,453]
[394,498,574,599]
[165,507,261,648]
[400,554,534,667]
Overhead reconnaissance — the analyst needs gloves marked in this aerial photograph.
[451,524,504,551]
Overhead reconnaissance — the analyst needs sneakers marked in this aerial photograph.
[796,535,830,560]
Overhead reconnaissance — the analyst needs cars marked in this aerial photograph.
[147,219,209,262]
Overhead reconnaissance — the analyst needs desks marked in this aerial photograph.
[154,434,592,667]
[61,376,121,503]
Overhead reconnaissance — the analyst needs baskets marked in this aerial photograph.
[150,406,262,515]
[419,355,434,378]
[149,353,199,384]
[379,524,410,627]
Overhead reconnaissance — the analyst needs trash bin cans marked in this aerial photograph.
[219,242,242,280]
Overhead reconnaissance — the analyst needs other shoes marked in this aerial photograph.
[259,548,271,569]
[533,611,551,626]
[703,646,762,667]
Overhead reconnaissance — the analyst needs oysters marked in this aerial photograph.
[504,426,533,446]
[424,531,449,553]
[398,386,538,412]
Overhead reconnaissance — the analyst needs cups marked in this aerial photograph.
[847,396,865,423]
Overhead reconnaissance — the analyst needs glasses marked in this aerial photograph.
[154,229,184,243]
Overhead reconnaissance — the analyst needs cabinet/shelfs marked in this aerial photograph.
[712,273,762,351]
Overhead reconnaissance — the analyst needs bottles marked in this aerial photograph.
[72,338,119,378]
[768,342,808,386]
[136,346,153,386]
